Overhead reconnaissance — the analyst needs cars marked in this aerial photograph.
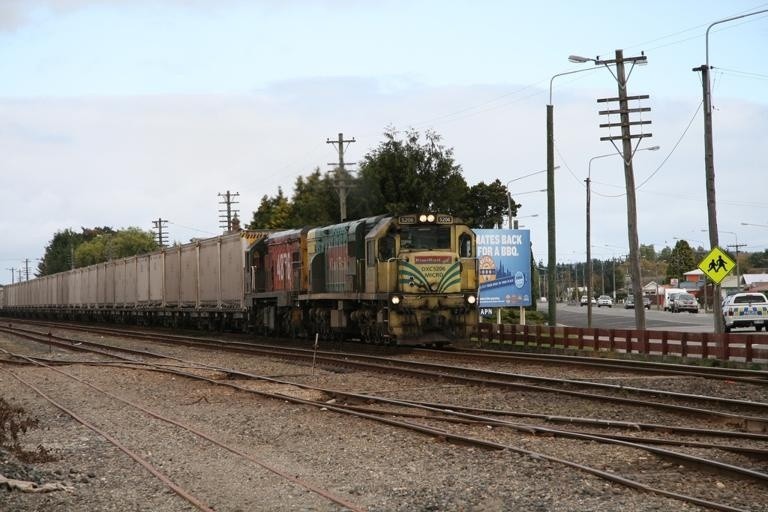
[722,292,768,332]
[643,298,651,310]
[581,295,596,306]
[673,294,699,313]
[597,295,614,307]
[623,295,635,308]
[539,296,548,303]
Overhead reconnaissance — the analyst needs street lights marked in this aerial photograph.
[585,144,660,325]
[505,164,561,230]
[569,54,656,354]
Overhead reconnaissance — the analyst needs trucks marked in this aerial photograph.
[663,288,687,311]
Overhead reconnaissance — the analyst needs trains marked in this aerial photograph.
[1,212,480,346]
